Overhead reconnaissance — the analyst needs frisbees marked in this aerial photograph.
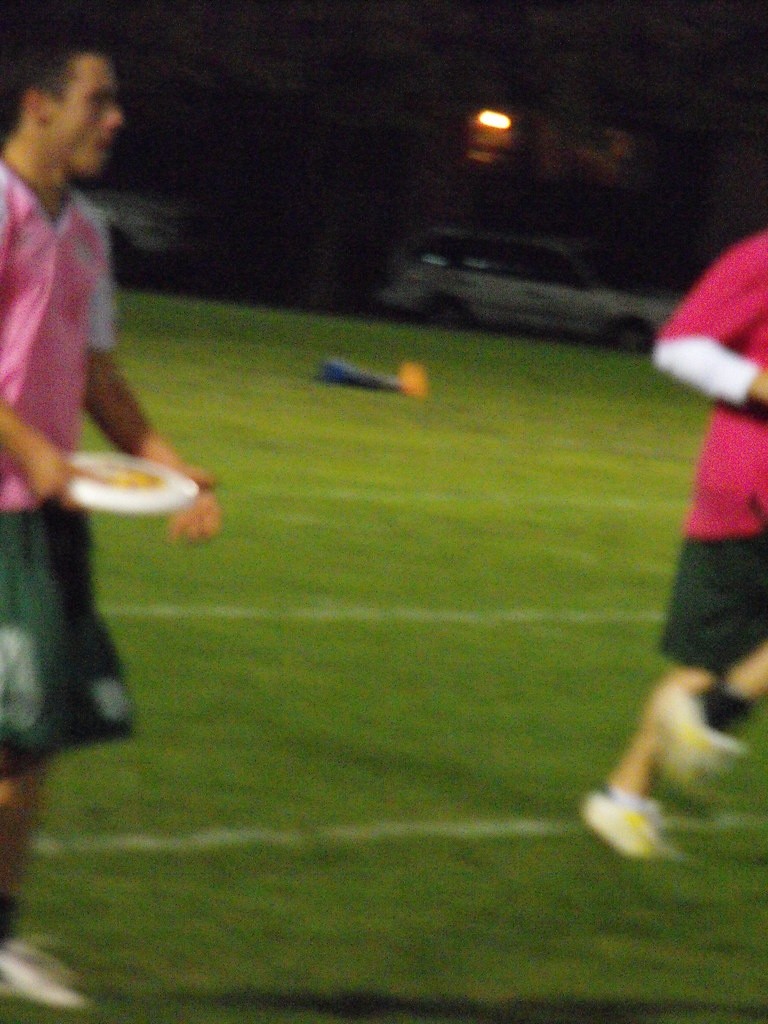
[46,454,198,519]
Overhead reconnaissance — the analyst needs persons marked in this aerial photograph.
[576,225,768,869]
[0,22,220,1010]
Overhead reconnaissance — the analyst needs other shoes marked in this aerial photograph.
[656,689,743,801]
[581,789,663,862]
[0,940,89,1013]
